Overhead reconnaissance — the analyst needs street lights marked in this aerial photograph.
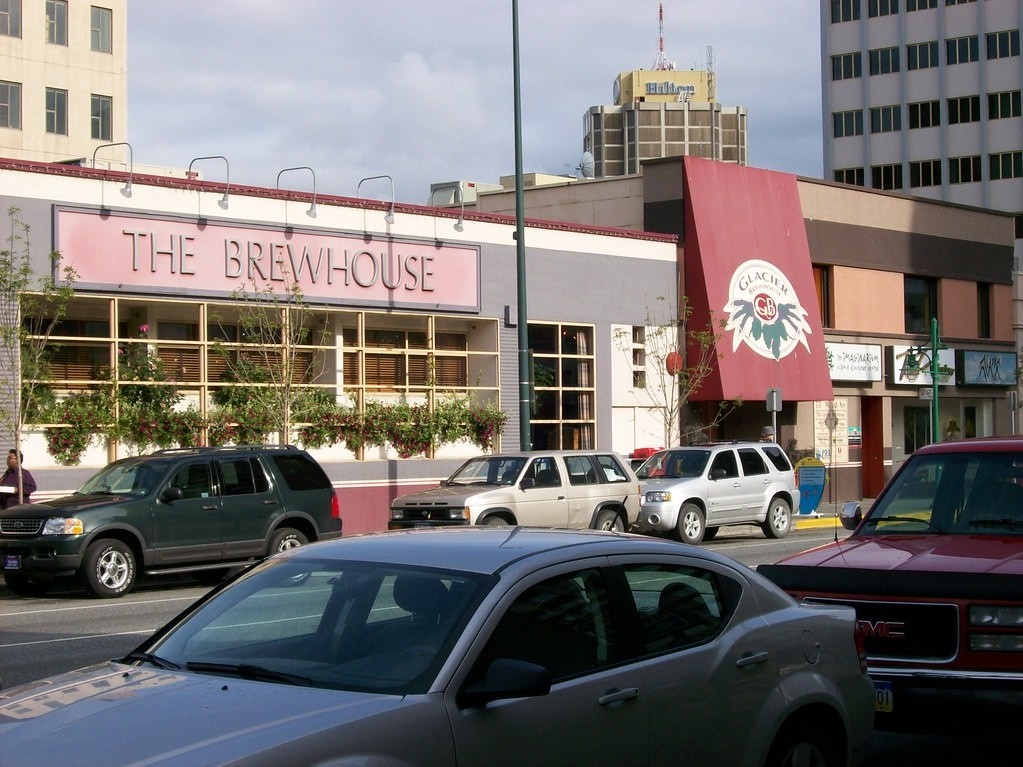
[906,317,948,443]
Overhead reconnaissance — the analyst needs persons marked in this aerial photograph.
[759,426,775,442]
[0,449,37,510]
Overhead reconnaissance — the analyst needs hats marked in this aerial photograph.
[761,426,774,436]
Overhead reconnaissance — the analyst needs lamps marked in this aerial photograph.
[432,186,464,232]
[277,167,317,218]
[357,175,394,223]
[189,156,230,209]
[93,142,133,198]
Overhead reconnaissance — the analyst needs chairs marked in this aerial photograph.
[959,481,1023,535]
[184,464,209,498]
[134,464,155,489]
[392,573,710,681]
[537,470,558,485]
[587,464,618,483]
[502,471,516,482]
[681,455,705,472]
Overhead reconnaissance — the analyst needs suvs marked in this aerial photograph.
[387,450,641,533]
[754,434,1022,767]
[0,444,343,597]
[634,438,800,546]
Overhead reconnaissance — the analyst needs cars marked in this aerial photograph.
[1,524,875,767]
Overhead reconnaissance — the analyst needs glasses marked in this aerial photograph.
[6,455,18,461]
